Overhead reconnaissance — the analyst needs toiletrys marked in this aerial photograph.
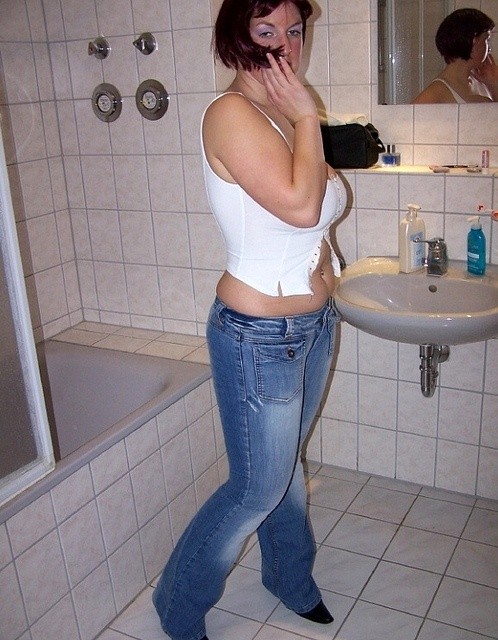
[398,204,427,274]
[468,204,486,277]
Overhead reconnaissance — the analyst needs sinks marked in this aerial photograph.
[335,270,497,316]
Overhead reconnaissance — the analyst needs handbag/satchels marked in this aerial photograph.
[321,123,385,168]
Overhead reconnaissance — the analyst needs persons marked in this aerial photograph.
[407,3,496,104]
[152,0,349,635]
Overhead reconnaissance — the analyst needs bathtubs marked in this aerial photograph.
[1,338,218,519]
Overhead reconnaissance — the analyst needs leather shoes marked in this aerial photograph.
[201,635,209,640]
[298,600,333,624]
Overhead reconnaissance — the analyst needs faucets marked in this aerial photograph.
[413,237,448,275]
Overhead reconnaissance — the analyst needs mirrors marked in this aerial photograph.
[378,0,498,104]
